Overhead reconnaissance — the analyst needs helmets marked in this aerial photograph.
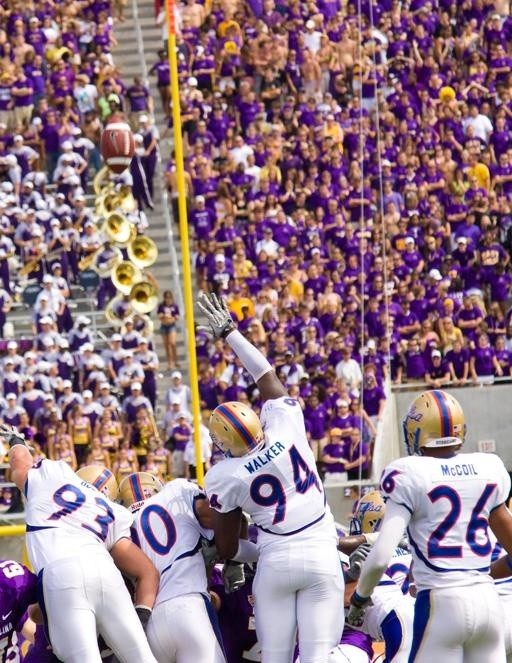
[117,471,163,512]
[349,490,386,537]
[77,463,118,502]
[403,389,466,455]
[208,400,265,456]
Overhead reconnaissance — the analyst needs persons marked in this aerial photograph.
[1,0,512,659]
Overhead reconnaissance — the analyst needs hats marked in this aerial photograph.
[405,237,414,244]
[4,350,182,405]
[330,428,342,436]
[215,253,226,262]
[444,298,453,306]
[7,262,147,352]
[429,269,442,280]
[381,159,391,167]
[188,76,198,87]
[195,195,204,203]
[1,115,149,237]
[431,350,441,358]
[457,237,467,244]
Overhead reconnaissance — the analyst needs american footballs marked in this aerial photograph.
[100,111,134,172]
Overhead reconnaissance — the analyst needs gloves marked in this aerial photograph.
[220,561,246,595]
[348,542,373,581]
[0,424,35,452]
[346,603,365,626]
[195,291,237,336]
